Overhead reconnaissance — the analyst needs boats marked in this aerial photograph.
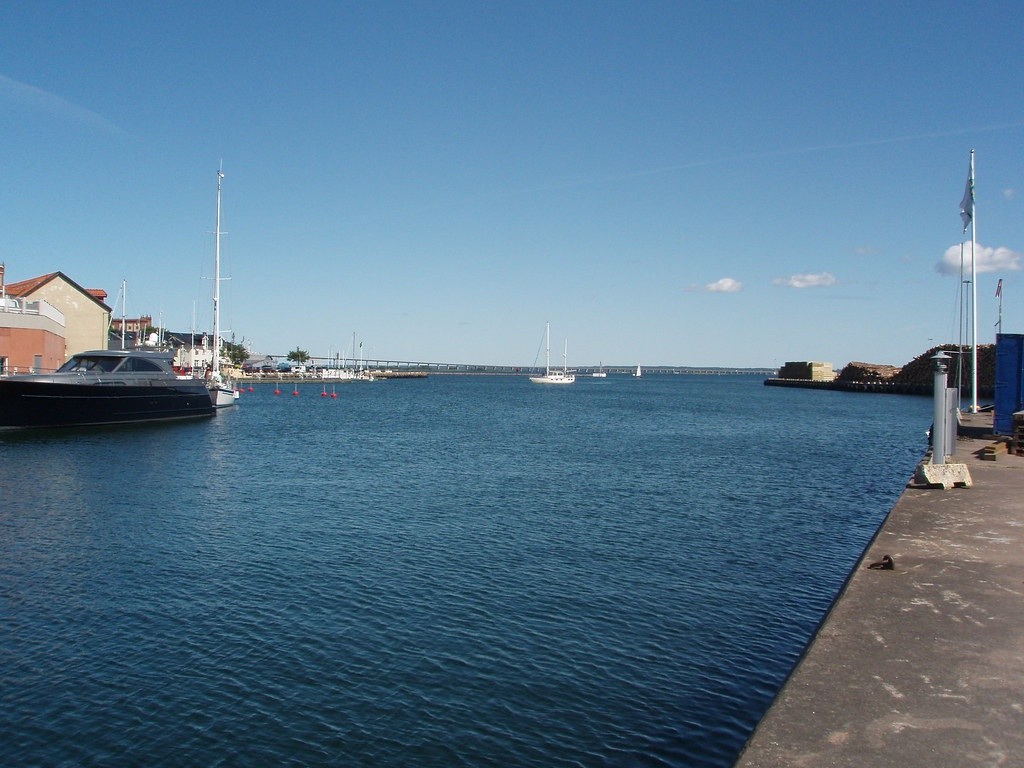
[0,346,217,431]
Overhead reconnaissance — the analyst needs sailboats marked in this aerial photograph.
[635,365,642,377]
[529,322,576,384]
[201,159,240,410]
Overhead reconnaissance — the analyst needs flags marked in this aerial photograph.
[959,160,975,234]
[995,279,1002,297]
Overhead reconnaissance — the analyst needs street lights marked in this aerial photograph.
[962,280,973,345]
